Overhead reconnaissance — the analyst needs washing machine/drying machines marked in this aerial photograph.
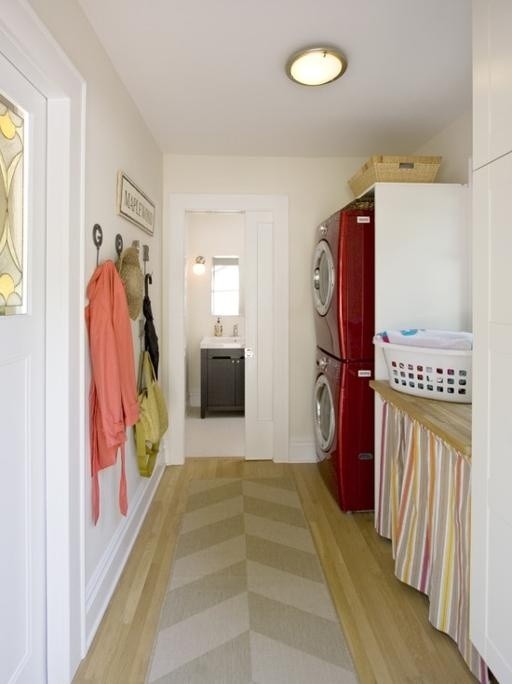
[310,198,374,359]
[310,347,374,513]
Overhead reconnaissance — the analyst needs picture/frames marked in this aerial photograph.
[119,173,157,236]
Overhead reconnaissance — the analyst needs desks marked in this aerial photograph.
[368,380,489,683]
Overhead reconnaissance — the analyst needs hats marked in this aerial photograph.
[115,248,146,322]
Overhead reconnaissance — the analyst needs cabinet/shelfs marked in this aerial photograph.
[470,152,511,681]
[208,349,244,413]
[470,2,512,174]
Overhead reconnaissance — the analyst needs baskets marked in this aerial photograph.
[373,334,473,404]
[347,155,443,200]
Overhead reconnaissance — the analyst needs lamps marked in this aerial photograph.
[285,45,348,88]
[192,255,208,275]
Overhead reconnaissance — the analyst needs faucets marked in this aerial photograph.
[233,323,238,336]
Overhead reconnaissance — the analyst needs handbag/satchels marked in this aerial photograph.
[138,352,170,445]
